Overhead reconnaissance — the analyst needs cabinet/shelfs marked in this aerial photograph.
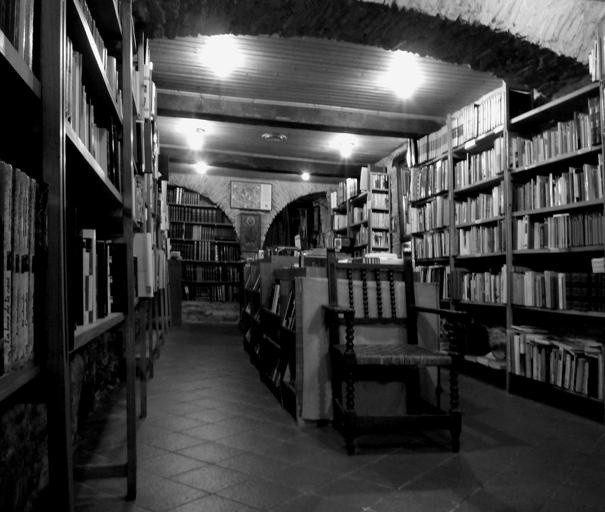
[293,163,409,255]
[407,37,604,426]
[242,248,421,429]
[1,1,171,510]
[164,184,243,303]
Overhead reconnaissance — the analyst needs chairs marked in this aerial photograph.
[325,248,468,455]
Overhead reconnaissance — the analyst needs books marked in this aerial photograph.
[330,166,403,265]
[2,0,170,510]
[244,247,296,387]
[168,187,243,303]
[400,34,605,399]
